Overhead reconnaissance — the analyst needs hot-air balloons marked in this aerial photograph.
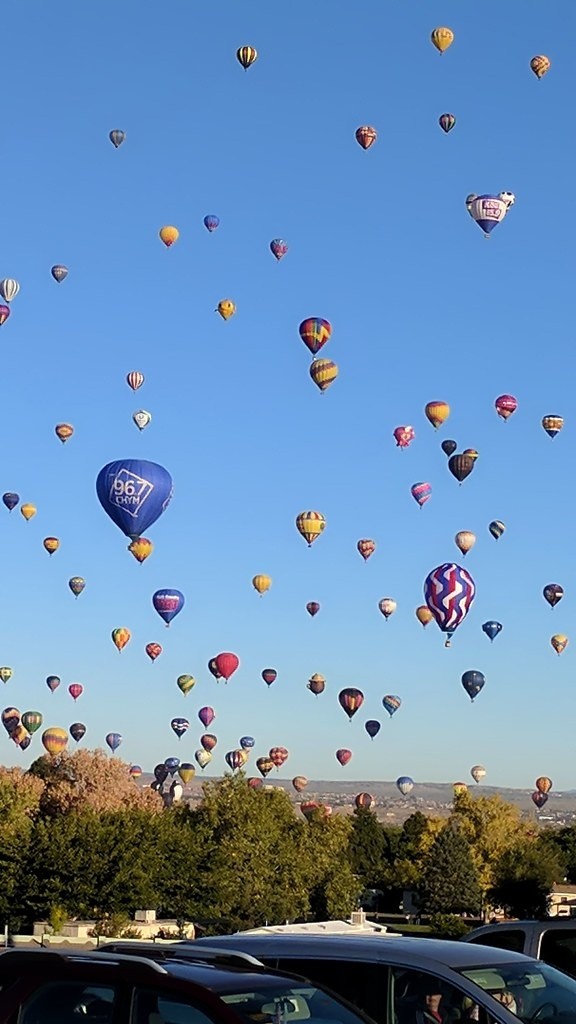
[0,26,566,819]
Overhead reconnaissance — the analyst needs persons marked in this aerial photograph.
[413,990,459,1024]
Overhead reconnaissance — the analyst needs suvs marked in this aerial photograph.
[458,920,576,1014]
[1,942,377,1023]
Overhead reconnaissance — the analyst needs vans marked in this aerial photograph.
[171,934,576,1024]
